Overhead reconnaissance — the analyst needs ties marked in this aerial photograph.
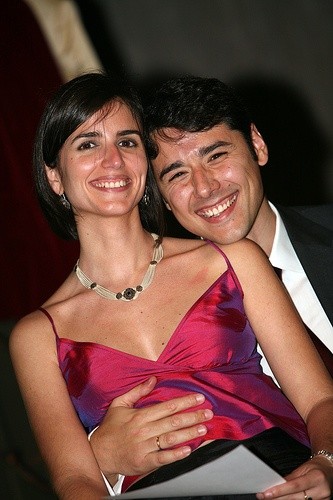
[272,266,333,381]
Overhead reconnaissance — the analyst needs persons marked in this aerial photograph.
[137,77,333,391]
[9,72,333,500]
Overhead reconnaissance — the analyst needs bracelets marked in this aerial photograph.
[309,449,333,464]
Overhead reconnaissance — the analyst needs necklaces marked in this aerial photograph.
[73,231,165,304]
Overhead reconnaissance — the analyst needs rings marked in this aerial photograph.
[304,490,313,500]
[156,435,164,450]
